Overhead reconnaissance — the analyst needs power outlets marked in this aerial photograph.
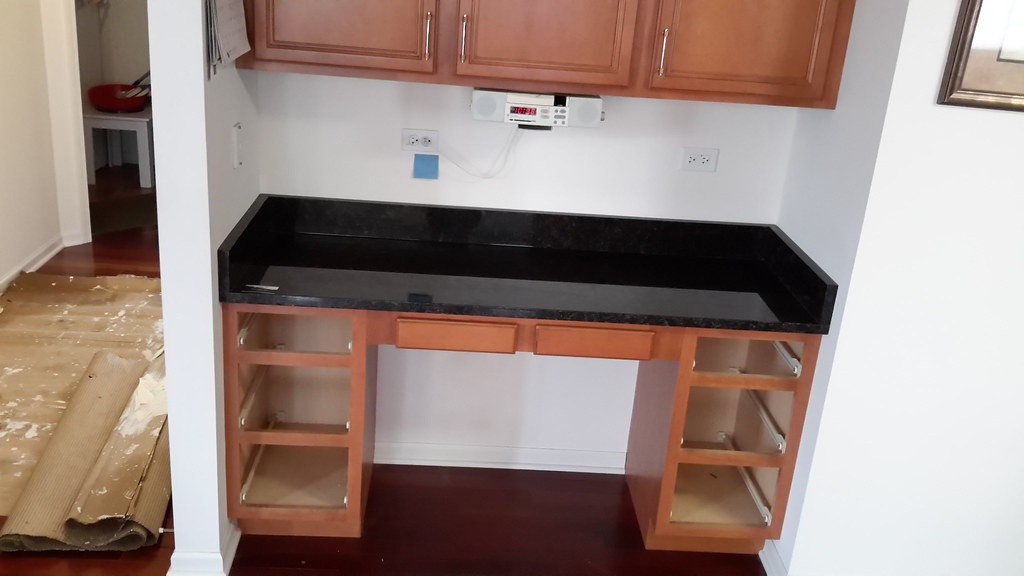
[681,147,720,172]
[400,128,441,152]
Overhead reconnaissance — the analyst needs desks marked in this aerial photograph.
[83,101,154,188]
[221,235,823,552]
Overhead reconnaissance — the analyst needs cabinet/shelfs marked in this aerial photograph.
[235,0,858,110]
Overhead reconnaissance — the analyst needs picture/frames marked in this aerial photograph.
[936,0,1024,112]
